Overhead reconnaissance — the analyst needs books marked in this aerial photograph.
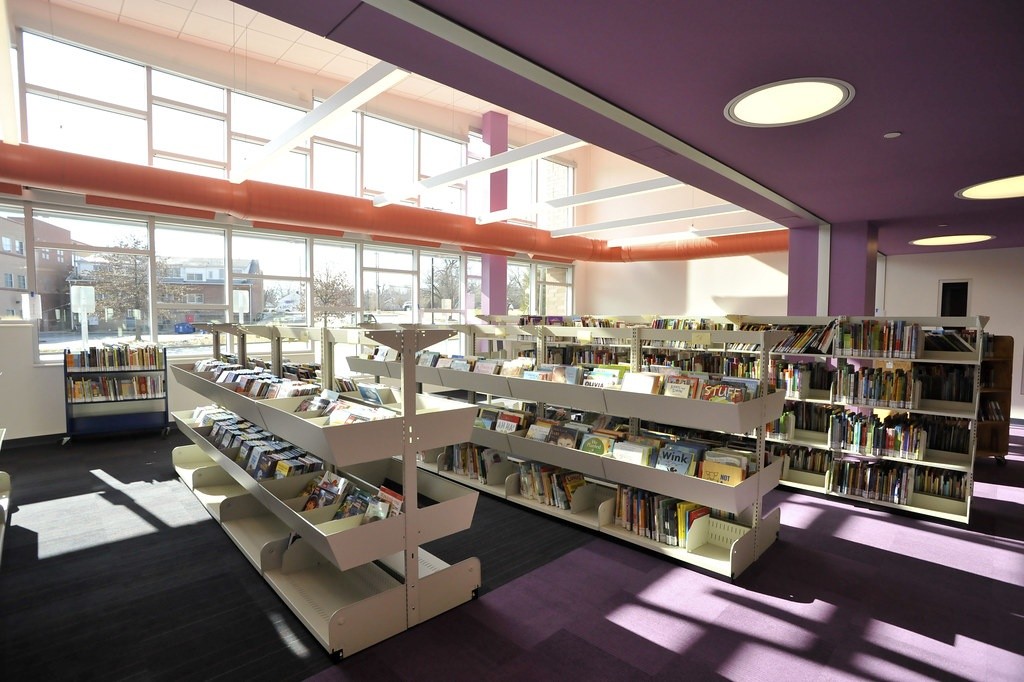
[192,352,404,525]
[65,340,165,403]
[361,316,1006,550]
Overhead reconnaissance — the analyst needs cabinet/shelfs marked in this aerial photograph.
[171,323,480,665]
[345,315,1015,581]
[56,346,171,436]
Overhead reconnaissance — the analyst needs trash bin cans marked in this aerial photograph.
[174,323,193,334]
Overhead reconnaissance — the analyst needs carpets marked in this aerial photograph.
[301,424,1024,682]
